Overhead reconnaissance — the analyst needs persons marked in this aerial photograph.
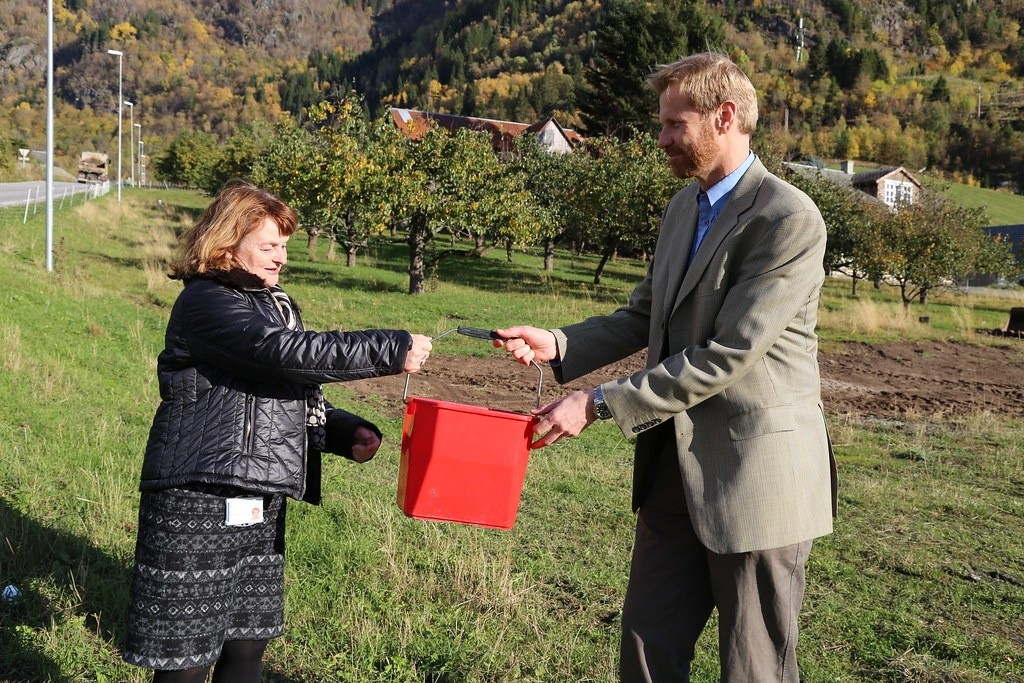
[493,52,838,683]
[124,181,433,683]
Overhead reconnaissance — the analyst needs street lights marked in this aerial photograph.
[134,123,143,187]
[137,140,144,187]
[108,49,123,203]
[124,100,134,187]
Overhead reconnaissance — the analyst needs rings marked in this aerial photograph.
[420,353,426,365]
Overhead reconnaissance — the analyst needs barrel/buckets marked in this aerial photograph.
[396,326,543,531]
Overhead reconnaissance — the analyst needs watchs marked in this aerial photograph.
[593,385,612,420]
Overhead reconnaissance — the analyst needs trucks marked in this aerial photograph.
[77,152,111,185]
